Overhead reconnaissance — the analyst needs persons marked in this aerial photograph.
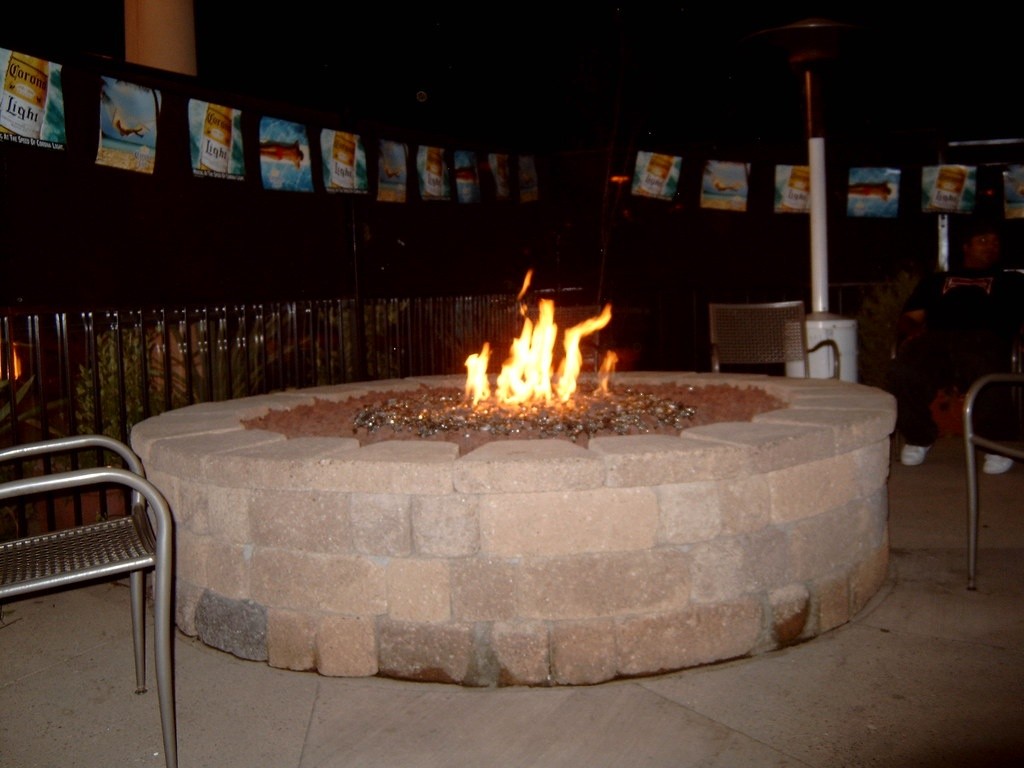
[883,223,1024,475]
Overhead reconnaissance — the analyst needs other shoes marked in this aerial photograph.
[901,443,930,466]
[982,454,1014,473]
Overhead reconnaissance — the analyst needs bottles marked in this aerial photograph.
[929,165,967,211]
[420,147,445,199]
[0,48,50,140]
[781,165,812,212]
[638,153,676,196]
[330,131,358,192]
[200,103,233,173]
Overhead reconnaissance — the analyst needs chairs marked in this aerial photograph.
[0,433,179,768]
[710,301,841,380]
[963,373,1024,590]
